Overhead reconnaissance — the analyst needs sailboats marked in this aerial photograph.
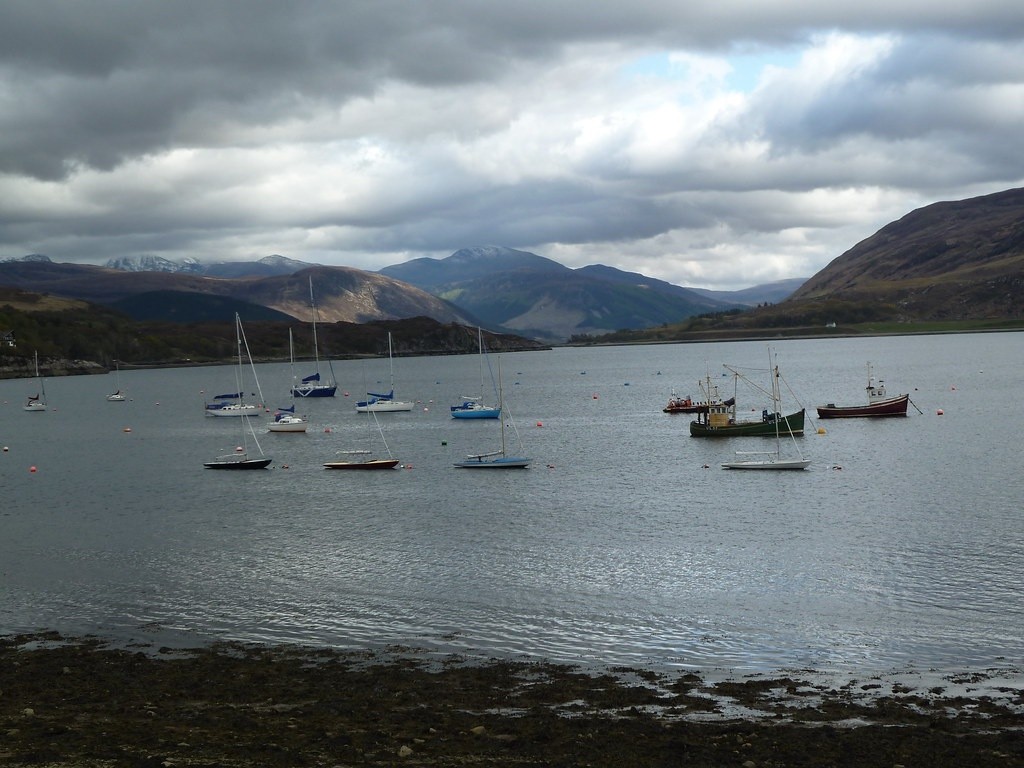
[451,326,501,418]
[106,360,125,402]
[721,347,812,469]
[22,350,48,410]
[355,332,414,412]
[689,345,805,436]
[290,275,338,397]
[203,312,265,416]
[266,327,308,431]
[662,359,735,412]
[203,366,272,469]
[453,354,534,467]
[816,361,910,418]
[322,363,399,469]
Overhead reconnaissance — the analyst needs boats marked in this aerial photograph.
[870,380,887,402]
